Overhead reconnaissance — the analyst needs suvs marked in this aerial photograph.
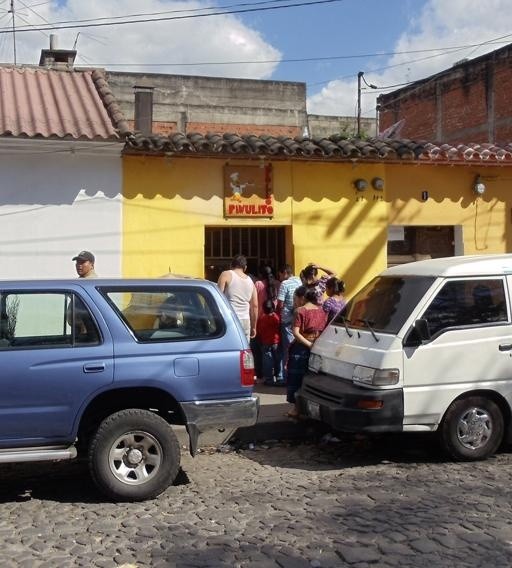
[1,278,259,500]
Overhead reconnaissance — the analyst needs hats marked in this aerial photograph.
[73,251,94,263]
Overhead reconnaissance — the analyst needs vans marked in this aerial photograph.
[294,253,512,462]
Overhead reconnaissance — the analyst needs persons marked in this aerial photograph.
[218,255,259,346]
[66,251,100,333]
[254,263,348,421]
